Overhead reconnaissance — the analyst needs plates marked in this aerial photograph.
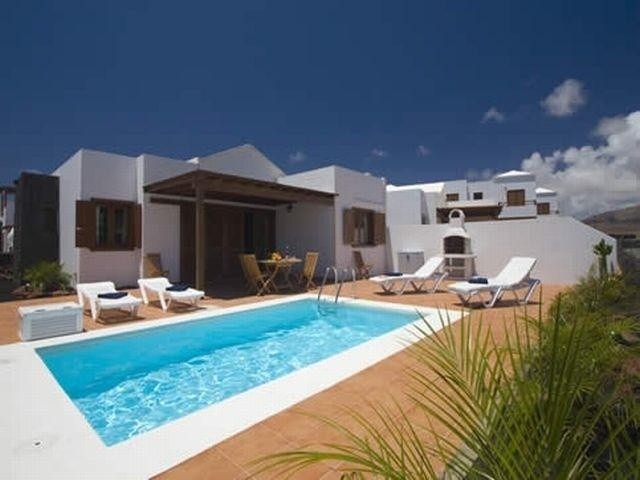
[16,301,83,342]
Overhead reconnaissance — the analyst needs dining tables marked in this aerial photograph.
[20,258,73,297]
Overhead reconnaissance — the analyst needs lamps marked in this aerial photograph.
[75,252,205,323]
[237,251,320,297]
[353,249,540,309]
